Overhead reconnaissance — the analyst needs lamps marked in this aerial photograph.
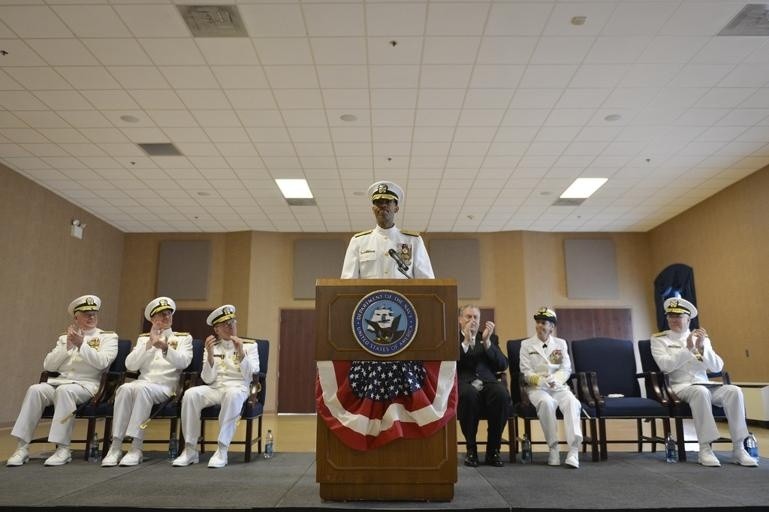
[70,220,86,239]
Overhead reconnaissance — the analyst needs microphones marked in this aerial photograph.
[387,248,409,271]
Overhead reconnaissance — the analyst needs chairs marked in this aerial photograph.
[133,339,202,460]
[31,339,131,461]
[571,337,675,461]
[507,337,599,464]
[638,340,747,463]
[203,337,270,463]
[458,334,516,463]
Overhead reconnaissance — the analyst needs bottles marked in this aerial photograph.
[745,431,760,463]
[519,434,533,464]
[263,429,274,459]
[90,432,100,464]
[166,432,178,462]
[665,432,677,465]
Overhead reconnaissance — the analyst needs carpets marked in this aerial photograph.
[0,451,769,512]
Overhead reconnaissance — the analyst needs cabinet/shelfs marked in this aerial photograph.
[732,382,769,422]
[314,279,459,503]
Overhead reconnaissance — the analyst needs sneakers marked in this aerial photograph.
[731,449,757,466]
[44,447,72,466]
[207,454,228,467]
[118,449,143,465]
[565,448,580,469]
[6,449,28,467]
[698,449,721,466]
[548,447,561,466]
[101,448,121,467]
[173,450,198,465]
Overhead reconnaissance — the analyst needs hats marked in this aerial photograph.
[368,182,404,205]
[663,298,697,320]
[534,307,557,325]
[207,304,235,325]
[144,296,176,321]
[68,295,101,316]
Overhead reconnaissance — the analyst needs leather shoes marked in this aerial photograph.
[486,450,503,467]
[466,448,478,467]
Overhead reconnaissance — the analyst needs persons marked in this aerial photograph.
[100,296,193,466]
[456,304,511,467]
[172,304,261,468]
[518,308,583,469]
[6,295,118,467]
[650,297,760,468]
[340,181,436,279]
